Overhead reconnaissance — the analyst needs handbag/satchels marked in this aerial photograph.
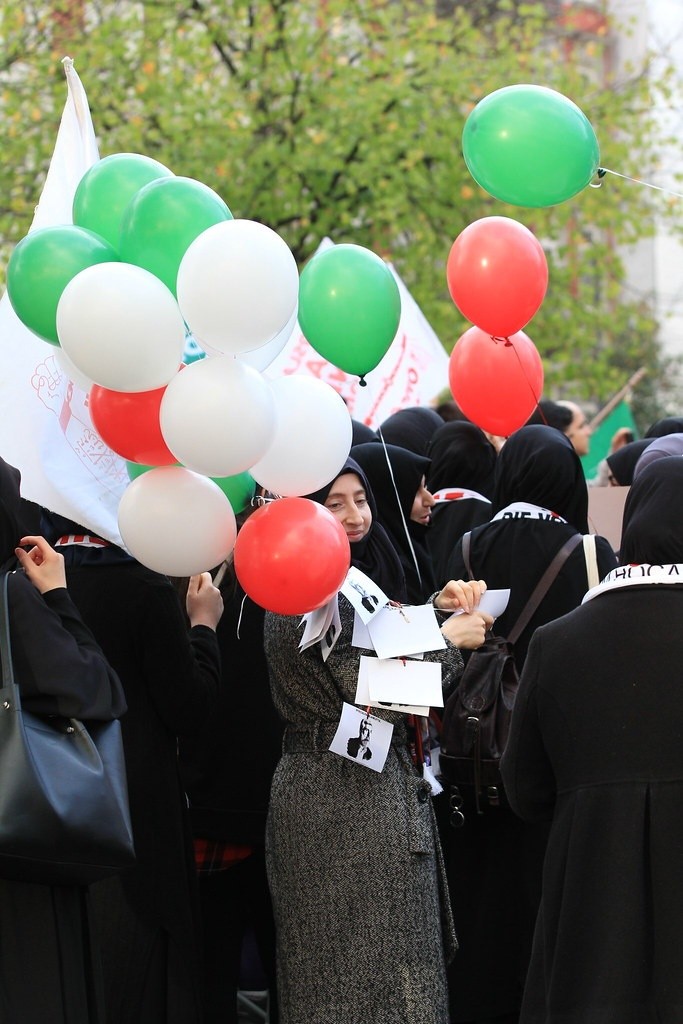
[0,571,138,870]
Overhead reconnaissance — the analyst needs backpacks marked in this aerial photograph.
[438,531,582,835]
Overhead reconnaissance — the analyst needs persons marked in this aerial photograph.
[596,417,683,486]
[508,436,683,1024]
[1,398,614,1024]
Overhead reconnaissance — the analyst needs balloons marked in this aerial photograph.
[117,175,232,299]
[447,217,548,345]
[249,377,354,498]
[91,361,189,467]
[297,241,401,375]
[462,83,600,208]
[4,224,120,347]
[446,325,543,438]
[117,463,237,577]
[124,457,256,515]
[71,153,174,251]
[176,217,298,356]
[157,358,277,478]
[236,496,352,617]
[55,264,186,395]
